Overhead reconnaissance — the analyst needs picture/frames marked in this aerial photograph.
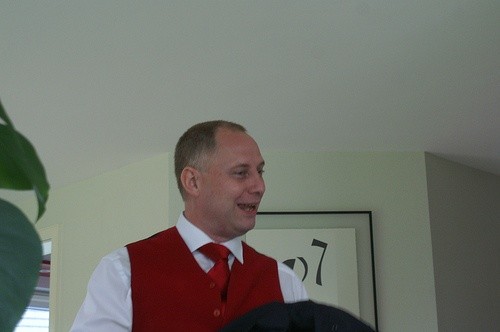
[245,210,379,332]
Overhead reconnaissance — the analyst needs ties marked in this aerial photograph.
[196,242,232,299]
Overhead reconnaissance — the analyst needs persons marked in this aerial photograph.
[68,120,309,332]
